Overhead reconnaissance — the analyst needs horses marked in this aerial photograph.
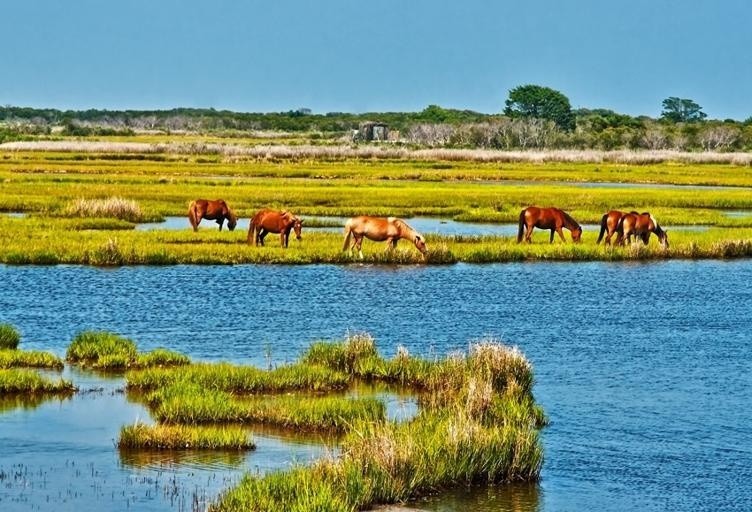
[188,197,239,232]
[614,210,670,249]
[342,215,428,260]
[517,206,583,244]
[245,208,305,248]
[596,208,651,247]
